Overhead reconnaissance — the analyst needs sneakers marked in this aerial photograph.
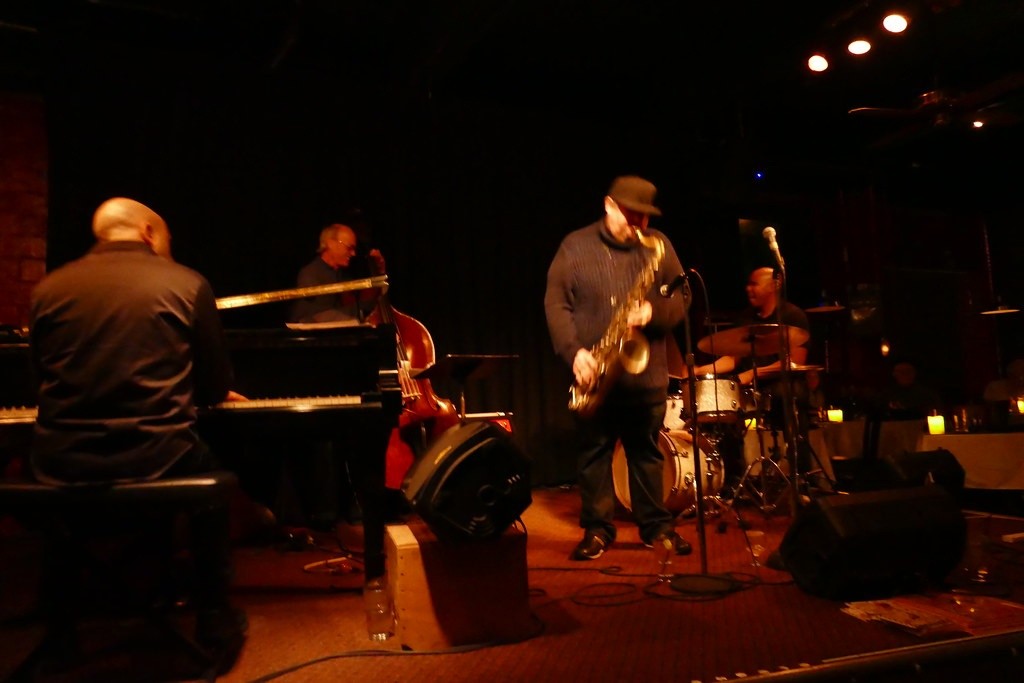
[573,532,607,560]
[647,532,692,555]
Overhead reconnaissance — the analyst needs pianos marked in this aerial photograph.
[2,274,405,580]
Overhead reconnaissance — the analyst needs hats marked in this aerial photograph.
[610,177,661,216]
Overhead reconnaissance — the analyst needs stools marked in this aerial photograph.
[0,472,239,683]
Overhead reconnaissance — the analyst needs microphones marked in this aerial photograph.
[659,269,693,298]
[763,227,784,270]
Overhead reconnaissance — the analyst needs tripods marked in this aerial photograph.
[674,264,838,535]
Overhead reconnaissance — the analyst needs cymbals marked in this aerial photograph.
[696,323,809,357]
[800,305,845,312]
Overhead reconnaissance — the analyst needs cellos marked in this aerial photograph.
[344,244,461,492]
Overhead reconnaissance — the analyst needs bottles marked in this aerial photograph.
[364,576,393,643]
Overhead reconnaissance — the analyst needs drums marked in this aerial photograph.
[609,372,822,516]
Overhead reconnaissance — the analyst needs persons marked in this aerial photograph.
[718,266,811,493]
[544,175,691,559]
[291,223,355,324]
[6,196,241,674]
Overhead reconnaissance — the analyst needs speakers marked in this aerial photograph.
[400,418,533,543]
[780,486,968,602]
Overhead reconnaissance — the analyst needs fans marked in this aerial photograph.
[849,71,1024,148]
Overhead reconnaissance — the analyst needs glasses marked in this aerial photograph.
[334,239,355,252]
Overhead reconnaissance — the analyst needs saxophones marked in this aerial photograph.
[568,222,666,415]
